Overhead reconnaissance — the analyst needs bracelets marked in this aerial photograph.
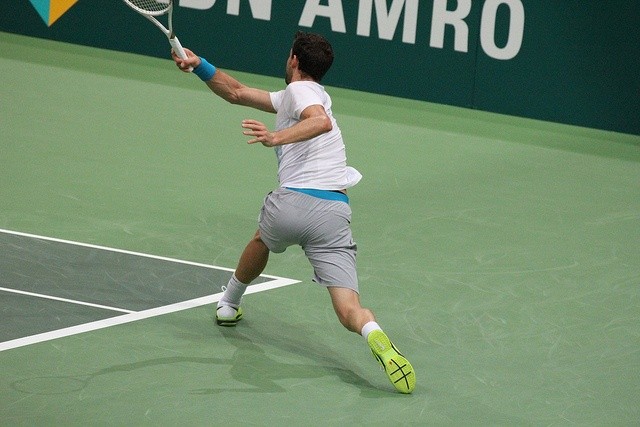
[191,55,217,82]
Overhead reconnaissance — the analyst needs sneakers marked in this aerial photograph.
[216,286,244,327]
[368,329,416,394]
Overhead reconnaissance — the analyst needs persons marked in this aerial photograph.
[169,30,417,394]
